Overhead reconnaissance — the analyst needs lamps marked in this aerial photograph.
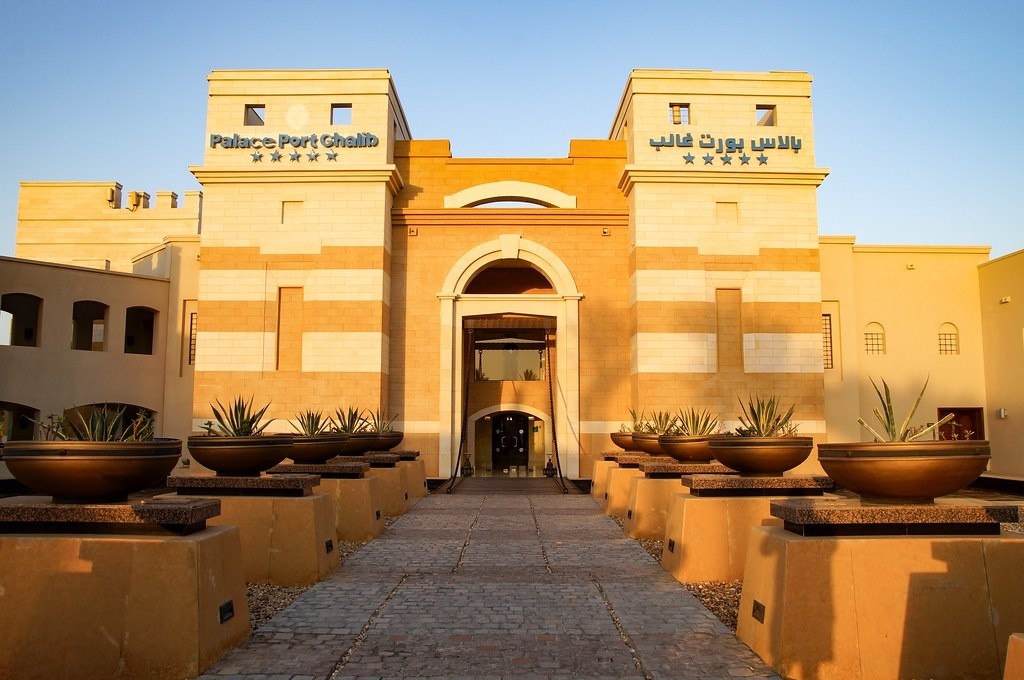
[106,187,114,208]
[126,191,138,212]
[999,408,1007,419]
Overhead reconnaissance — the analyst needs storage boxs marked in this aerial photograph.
[509,465,517,470]
[519,466,526,470]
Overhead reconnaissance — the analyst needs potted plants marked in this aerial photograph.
[632,408,664,455]
[610,423,632,451]
[358,407,403,452]
[1,400,183,504]
[186,395,294,475]
[658,406,734,465]
[325,403,380,456]
[816,373,993,505]
[273,408,350,463]
[706,392,814,477]
